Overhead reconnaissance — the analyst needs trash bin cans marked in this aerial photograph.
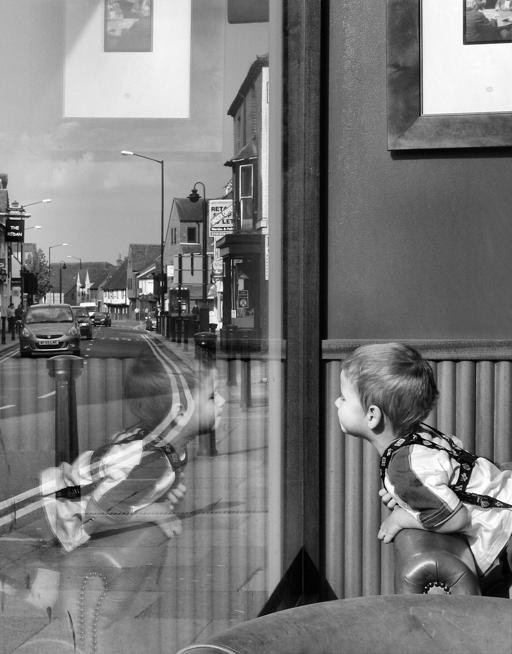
[194,332,217,369]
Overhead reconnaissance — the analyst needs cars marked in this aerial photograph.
[14,302,114,360]
[146,312,164,332]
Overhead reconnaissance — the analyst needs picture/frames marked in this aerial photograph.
[384,0,512,152]
[30,1,229,158]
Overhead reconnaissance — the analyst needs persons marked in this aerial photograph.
[135,305,141,320]
[333,340,511,598]
[145,305,149,317]
[6,302,16,333]
[1,336,227,596]
[15,303,24,320]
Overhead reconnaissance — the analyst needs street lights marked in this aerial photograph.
[19,196,56,313]
[187,179,212,335]
[66,253,83,269]
[16,225,41,262]
[46,241,69,304]
[58,258,68,304]
[121,148,167,317]
